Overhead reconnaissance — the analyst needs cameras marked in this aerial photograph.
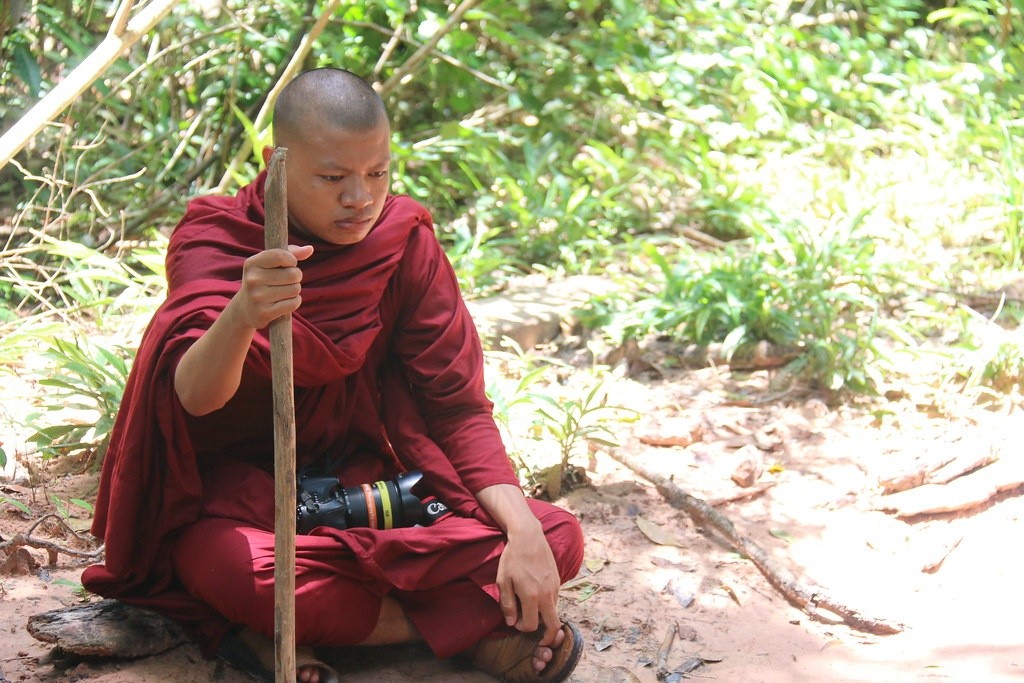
[296,469,432,536]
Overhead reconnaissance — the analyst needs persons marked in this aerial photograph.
[81,67,583,683]
